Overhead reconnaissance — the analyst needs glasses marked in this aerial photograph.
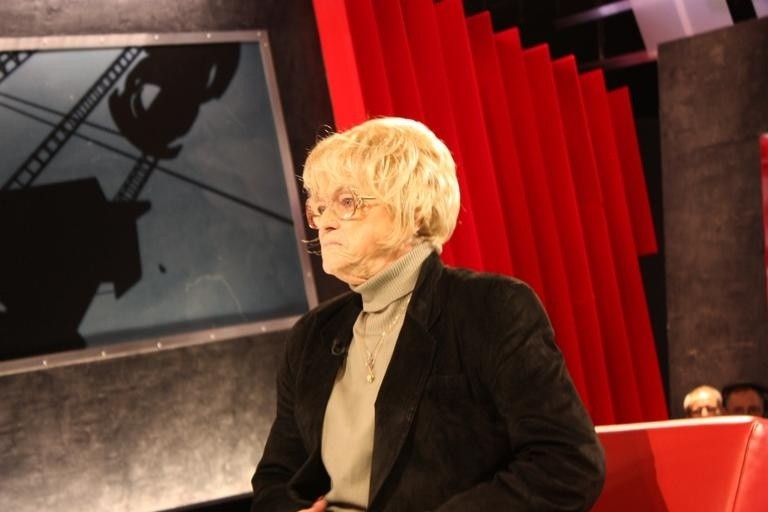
[305,187,379,229]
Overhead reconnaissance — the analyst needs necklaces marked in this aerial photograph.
[356,294,413,384]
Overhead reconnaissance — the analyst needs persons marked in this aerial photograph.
[721,382,768,418]
[249,116,607,512]
[683,384,723,417]
